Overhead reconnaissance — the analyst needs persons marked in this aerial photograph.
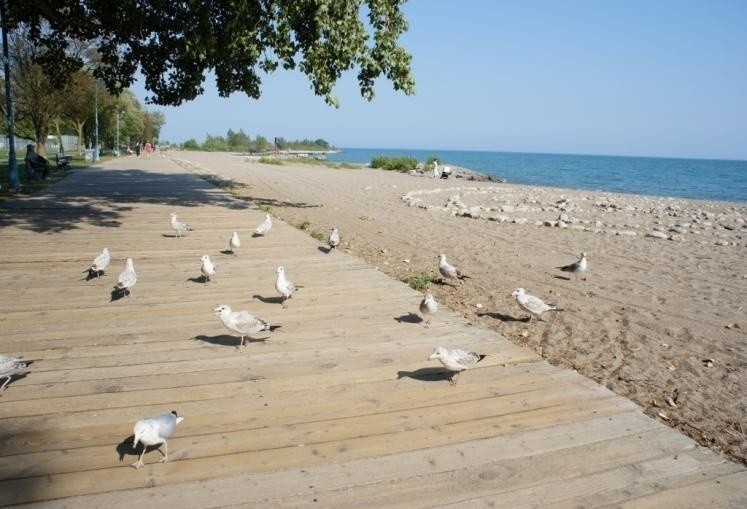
[432,157,439,181]
[126,139,155,158]
[26,144,53,183]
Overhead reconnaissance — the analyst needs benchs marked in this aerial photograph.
[55,153,72,171]
[24,158,49,183]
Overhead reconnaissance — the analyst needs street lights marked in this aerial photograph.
[115,110,126,154]
[91,72,103,163]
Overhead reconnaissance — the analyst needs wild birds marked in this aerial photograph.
[213,305,282,348]
[419,292,439,327]
[253,213,274,237]
[229,231,241,257]
[428,347,490,387]
[436,253,470,285]
[201,255,218,282]
[113,257,137,297]
[132,410,185,470]
[82,247,110,279]
[327,227,340,251]
[512,288,565,323]
[170,212,193,238]
[555,251,588,280]
[0,353,35,393]
[275,266,304,303]
